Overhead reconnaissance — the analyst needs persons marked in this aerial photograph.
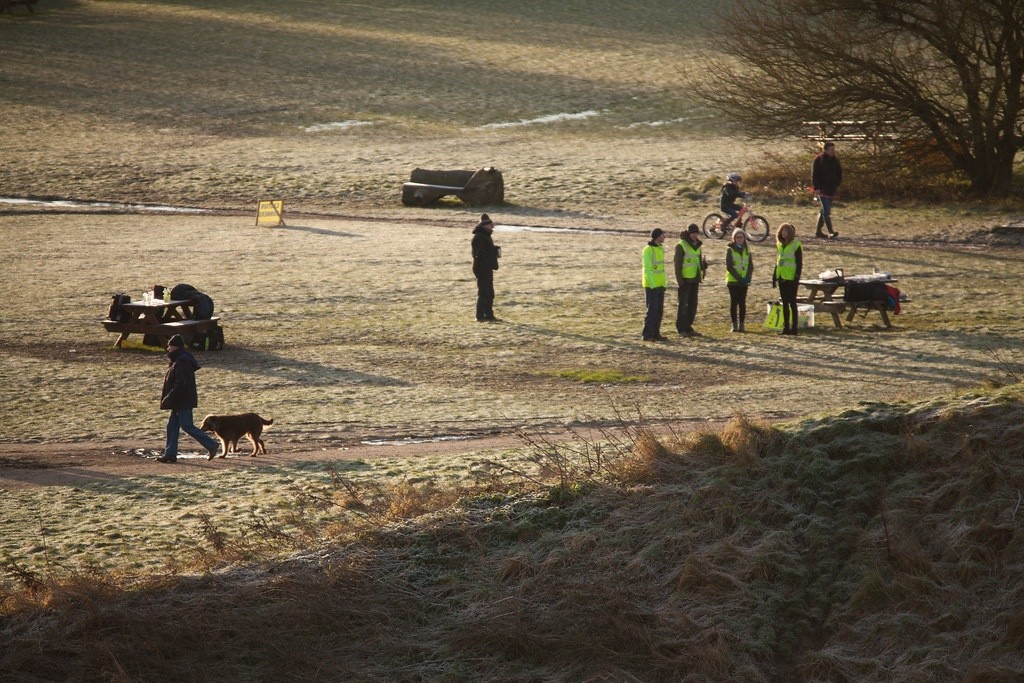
[726,228,754,333]
[772,222,802,335]
[674,224,708,338]
[156,334,218,463]
[471,214,502,323]
[812,142,842,239]
[720,173,749,233]
[642,228,668,341]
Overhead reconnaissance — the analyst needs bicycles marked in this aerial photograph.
[703,194,769,244]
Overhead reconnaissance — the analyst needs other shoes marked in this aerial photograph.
[783,326,790,333]
[828,231,839,239]
[720,223,727,233]
[688,328,701,336]
[738,324,744,332]
[680,330,691,337]
[642,334,668,340]
[815,232,828,238]
[790,328,798,335]
[733,324,737,332]
[476,317,490,322]
[486,314,503,322]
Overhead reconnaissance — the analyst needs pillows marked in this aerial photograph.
[463,167,494,190]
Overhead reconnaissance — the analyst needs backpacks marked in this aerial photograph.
[106,292,131,321]
[190,325,225,351]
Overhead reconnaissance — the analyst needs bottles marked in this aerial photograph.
[163,288,171,301]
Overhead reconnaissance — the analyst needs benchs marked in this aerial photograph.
[802,134,892,140]
[101,316,221,336]
[779,295,911,307]
[402,166,504,204]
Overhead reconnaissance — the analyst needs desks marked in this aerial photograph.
[803,121,898,147]
[799,279,899,329]
[113,298,193,349]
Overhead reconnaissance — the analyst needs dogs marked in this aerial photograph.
[199,413,274,459]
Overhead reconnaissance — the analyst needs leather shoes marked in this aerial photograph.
[207,442,219,461]
[157,457,176,463]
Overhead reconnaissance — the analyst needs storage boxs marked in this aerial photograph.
[767,302,814,329]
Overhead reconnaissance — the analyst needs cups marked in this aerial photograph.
[496,246,502,258]
[143,291,154,303]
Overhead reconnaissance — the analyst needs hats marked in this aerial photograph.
[732,228,746,242]
[480,213,492,224]
[688,223,699,233]
[651,227,666,240]
[168,333,184,346]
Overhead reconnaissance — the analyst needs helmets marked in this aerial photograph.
[726,173,741,182]
